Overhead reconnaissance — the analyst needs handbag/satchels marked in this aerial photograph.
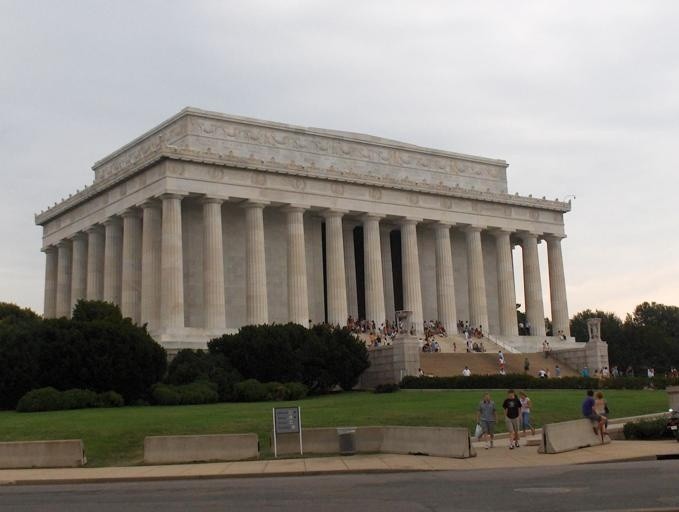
[604,406,609,414]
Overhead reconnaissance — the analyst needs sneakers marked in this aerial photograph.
[509,440,520,449]
[484,441,495,448]
[593,426,609,435]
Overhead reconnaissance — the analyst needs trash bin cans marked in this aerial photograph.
[338,431,355,455]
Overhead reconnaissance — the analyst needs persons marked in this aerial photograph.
[477,392,499,449]
[346,315,486,352]
[502,389,522,450]
[581,390,611,434]
[520,390,536,435]
[417,367,424,378]
[463,365,472,376]
[594,391,608,428]
[578,364,678,380]
[518,319,567,350]
[496,350,562,379]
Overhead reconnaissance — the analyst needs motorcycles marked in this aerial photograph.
[665,408,679,442]
